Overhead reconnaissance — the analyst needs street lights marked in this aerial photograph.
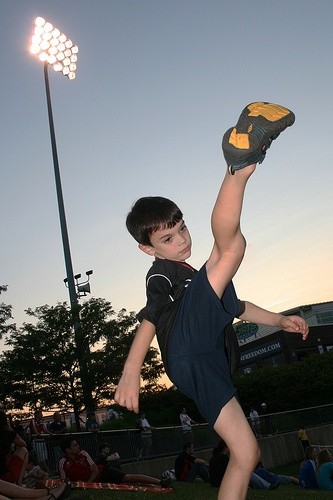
[29,15,104,455]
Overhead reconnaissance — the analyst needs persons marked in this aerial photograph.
[108,406,115,421]
[260,403,275,436]
[29,411,54,476]
[248,407,262,440]
[13,419,24,437]
[138,411,156,460]
[317,448,333,490]
[175,442,211,484]
[254,461,305,490]
[0,410,29,488]
[299,424,312,456]
[7,415,14,429]
[299,445,319,490]
[49,412,70,473]
[0,479,71,500]
[178,407,199,455]
[95,441,123,471]
[113,102,310,499]
[59,438,167,490]
[208,440,307,491]
[86,412,102,457]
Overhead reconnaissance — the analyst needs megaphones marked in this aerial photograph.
[79,282,91,294]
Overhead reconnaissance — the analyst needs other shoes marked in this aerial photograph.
[269,480,280,491]
[47,481,71,500]
[160,477,170,488]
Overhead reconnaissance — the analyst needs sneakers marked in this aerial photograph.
[222,102,295,175]
